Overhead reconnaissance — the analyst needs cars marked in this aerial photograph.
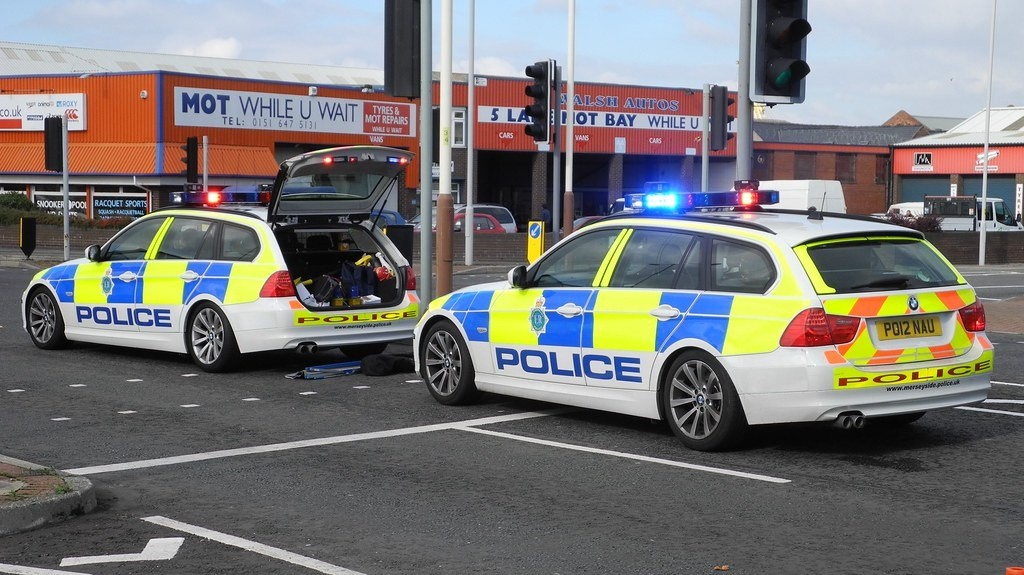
[560,215,608,232]
[412,212,506,233]
[867,202,925,226]
[369,209,407,229]
[411,181,994,454]
[22,144,424,374]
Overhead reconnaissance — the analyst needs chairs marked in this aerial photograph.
[307,234,333,251]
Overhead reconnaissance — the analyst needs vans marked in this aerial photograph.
[700,179,847,216]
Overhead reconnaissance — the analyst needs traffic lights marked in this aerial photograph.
[750,0,812,104]
[711,85,734,152]
[180,136,198,183]
[525,61,551,144]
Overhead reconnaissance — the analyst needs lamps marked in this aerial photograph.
[349,83,374,94]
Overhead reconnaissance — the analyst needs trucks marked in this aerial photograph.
[922,193,1024,233]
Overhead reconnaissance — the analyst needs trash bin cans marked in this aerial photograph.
[386,224,415,268]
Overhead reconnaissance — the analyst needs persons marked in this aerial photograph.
[541,204,550,232]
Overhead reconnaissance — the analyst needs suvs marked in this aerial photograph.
[404,203,519,233]
